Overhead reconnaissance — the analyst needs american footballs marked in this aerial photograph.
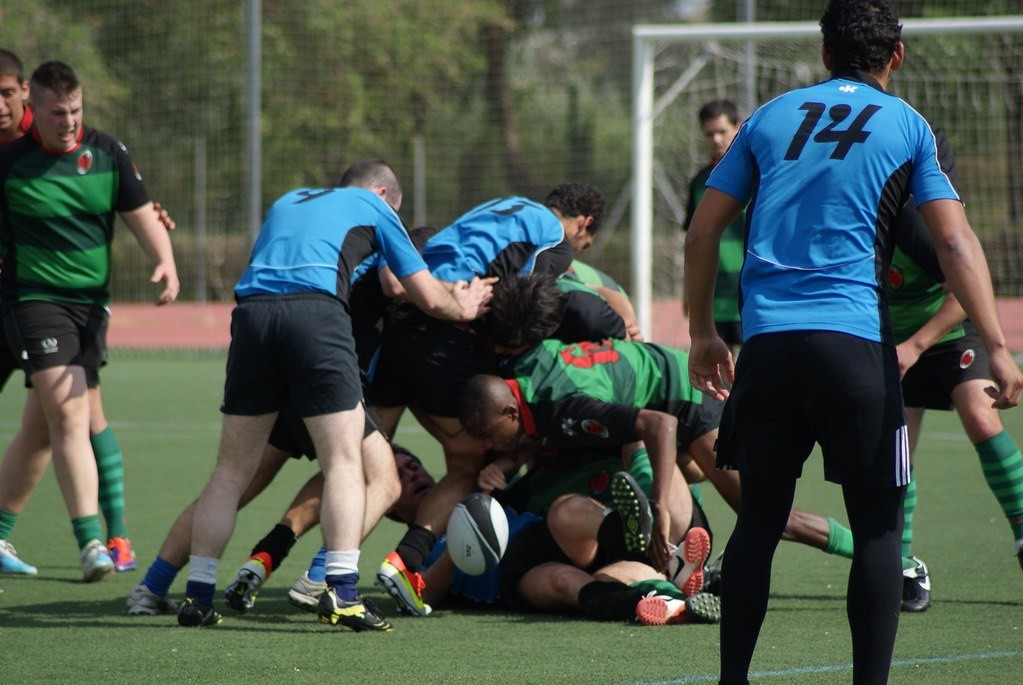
[445,492,510,578]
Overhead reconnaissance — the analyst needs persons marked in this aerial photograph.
[885,123,1023,570]
[128,181,930,625]
[174,160,494,628]
[685,98,751,380]
[682,0,1023,685]
[0,48,175,571]
[0,60,181,578]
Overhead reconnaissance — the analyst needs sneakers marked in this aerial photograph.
[224,552,272,616]
[611,471,654,553]
[376,551,432,617]
[666,527,710,598]
[901,554,931,613]
[125,585,179,616]
[106,535,137,572]
[287,569,329,613]
[0,539,38,575]
[79,538,114,581]
[178,596,223,627]
[684,591,721,623]
[319,586,394,633]
[635,590,690,626]
